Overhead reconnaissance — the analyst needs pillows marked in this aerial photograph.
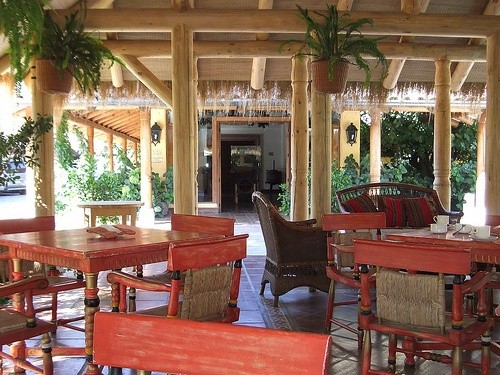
[340,191,377,214]
[377,195,408,228]
[402,197,435,229]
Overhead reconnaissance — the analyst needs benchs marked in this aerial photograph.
[336,183,464,229]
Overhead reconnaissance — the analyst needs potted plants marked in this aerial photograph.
[279,3,389,100]
[0,0,128,102]
[155,181,168,218]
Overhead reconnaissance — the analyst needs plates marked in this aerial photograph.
[471,235,498,242]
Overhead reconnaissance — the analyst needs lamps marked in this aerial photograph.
[151,122,162,146]
[345,123,358,146]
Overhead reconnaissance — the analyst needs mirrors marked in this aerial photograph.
[230,145,261,168]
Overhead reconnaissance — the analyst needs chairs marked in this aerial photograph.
[0,191,500,375]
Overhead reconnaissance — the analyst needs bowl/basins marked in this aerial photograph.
[430,224,447,233]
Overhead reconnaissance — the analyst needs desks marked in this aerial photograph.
[386,227,500,264]
[0,225,225,375]
[76,201,144,227]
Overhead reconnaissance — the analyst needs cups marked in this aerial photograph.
[455,223,472,234]
[433,215,450,225]
[472,225,491,239]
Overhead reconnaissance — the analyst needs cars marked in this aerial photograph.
[0,157,26,194]
